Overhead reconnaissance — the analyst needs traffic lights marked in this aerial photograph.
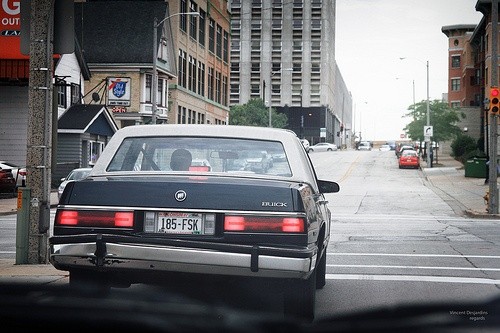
[489,88,500,115]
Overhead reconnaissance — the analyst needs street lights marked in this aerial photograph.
[399,57,430,168]
[353,101,368,143]
[151,11,200,125]
[396,77,416,149]
[269,68,294,126]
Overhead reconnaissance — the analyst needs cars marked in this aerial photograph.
[58,168,93,200]
[49,124,340,326]
[379,145,392,152]
[189,150,287,173]
[309,142,337,152]
[399,150,420,169]
[358,142,371,150]
[0,160,27,193]
[389,144,414,159]
[300,138,309,153]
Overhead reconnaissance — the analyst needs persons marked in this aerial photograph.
[170,148,192,171]
[355,141,359,151]
